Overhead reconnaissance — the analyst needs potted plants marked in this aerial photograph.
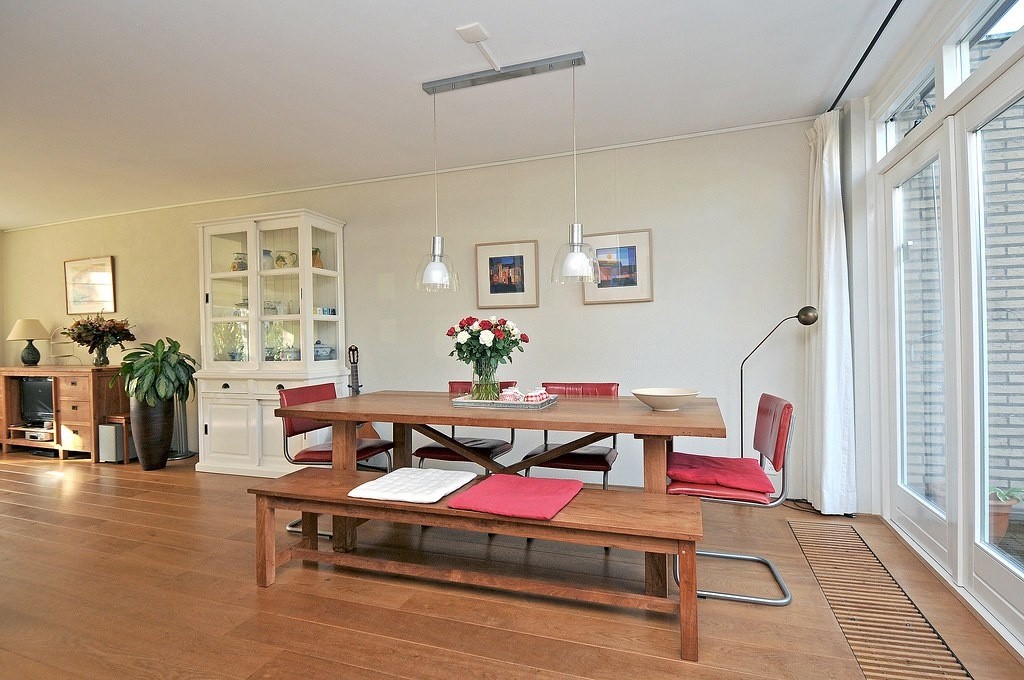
[109,337,201,471]
[989,486,1024,544]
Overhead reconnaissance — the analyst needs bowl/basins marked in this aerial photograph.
[226,301,336,361]
[630,387,702,411]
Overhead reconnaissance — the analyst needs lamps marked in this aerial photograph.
[414,89,461,293]
[6,319,50,366]
[741,306,818,458]
[552,60,600,285]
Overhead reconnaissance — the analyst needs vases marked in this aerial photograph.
[473,358,501,400]
[92,347,109,367]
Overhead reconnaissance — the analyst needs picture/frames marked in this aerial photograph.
[475,240,539,309]
[64,255,116,316]
[582,228,654,305]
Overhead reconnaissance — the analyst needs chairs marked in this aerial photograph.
[666,392,795,607]
[412,381,517,537]
[278,383,395,539]
[522,383,619,551]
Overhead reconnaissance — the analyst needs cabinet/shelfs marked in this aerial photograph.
[0,366,130,464]
[43,327,82,367]
[192,208,352,480]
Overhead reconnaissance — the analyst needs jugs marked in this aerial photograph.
[274,251,297,269]
[262,249,275,269]
[312,248,323,269]
[230,253,248,271]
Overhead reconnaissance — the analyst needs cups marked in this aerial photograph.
[500,385,550,404]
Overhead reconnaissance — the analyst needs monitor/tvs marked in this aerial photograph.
[20,377,53,427]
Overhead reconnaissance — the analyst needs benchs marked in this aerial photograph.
[247,467,704,661]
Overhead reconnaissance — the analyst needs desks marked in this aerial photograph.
[274,390,726,599]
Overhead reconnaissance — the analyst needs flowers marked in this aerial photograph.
[60,308,136,355]
[446,316,530,398]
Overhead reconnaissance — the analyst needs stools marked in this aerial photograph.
[103,413,132,464]
